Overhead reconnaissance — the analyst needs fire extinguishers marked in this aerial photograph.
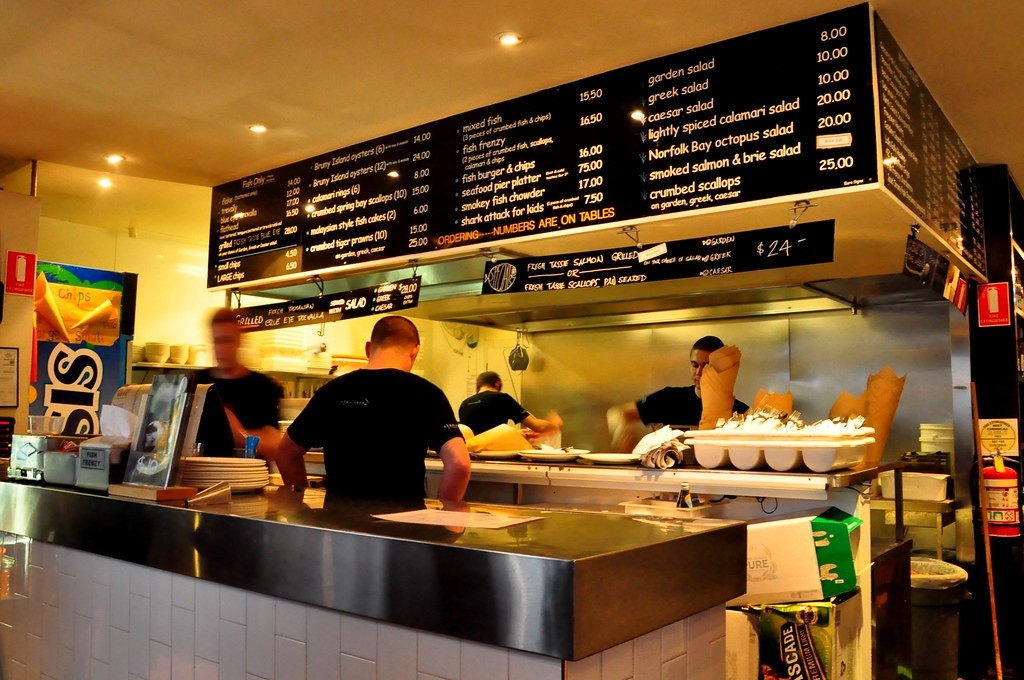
[978,454,1022,537]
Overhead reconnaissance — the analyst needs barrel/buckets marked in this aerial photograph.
[918,423,954,478]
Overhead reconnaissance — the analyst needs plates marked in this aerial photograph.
[471,451,519,459]
[580,453,640,464]
[181,456,270,493]
[517,449,591,462]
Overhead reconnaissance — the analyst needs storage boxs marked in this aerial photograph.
[618,500,724,518]
[726,586,863,680]
[878,470,950,501]
[726,506,874,607]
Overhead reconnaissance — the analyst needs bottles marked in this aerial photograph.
[677,481,694,509]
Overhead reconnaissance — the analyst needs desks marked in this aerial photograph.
[871,499,956,560]
[132,361,337,379]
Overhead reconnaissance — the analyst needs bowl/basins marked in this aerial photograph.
[133,342,188,363]
[29,415,65,435]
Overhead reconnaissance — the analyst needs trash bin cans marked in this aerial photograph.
[910,557,969,680]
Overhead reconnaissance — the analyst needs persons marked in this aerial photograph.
[193,307,284,474]
[605,336,750,455]
[458,371,564,435]
[274,316,471,499]
[269,486,471,544]
